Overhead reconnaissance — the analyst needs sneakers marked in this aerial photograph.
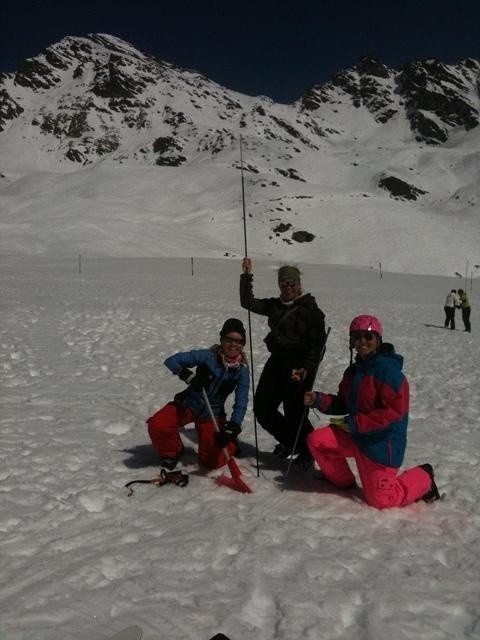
[280,454,316,475]
[416,463,441,503]
[161,447,185,471]
[273,442,292,461]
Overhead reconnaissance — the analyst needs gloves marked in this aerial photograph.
[179,368,208,394]
[214,420,241,449]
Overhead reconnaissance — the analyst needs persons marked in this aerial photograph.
[302,314,440,509]
[146,317,250,469]
[239,256,327,456]
[457,289,471,331]
[443,289,461,330]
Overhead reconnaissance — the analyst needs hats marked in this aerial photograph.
[220,318,246,338]
[278,266,301,285]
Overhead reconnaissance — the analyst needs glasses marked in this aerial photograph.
[280,281,296,288]
[223,336,244,345]
[354,333,373,342]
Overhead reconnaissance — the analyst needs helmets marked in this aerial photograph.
[349,315,383,338]
